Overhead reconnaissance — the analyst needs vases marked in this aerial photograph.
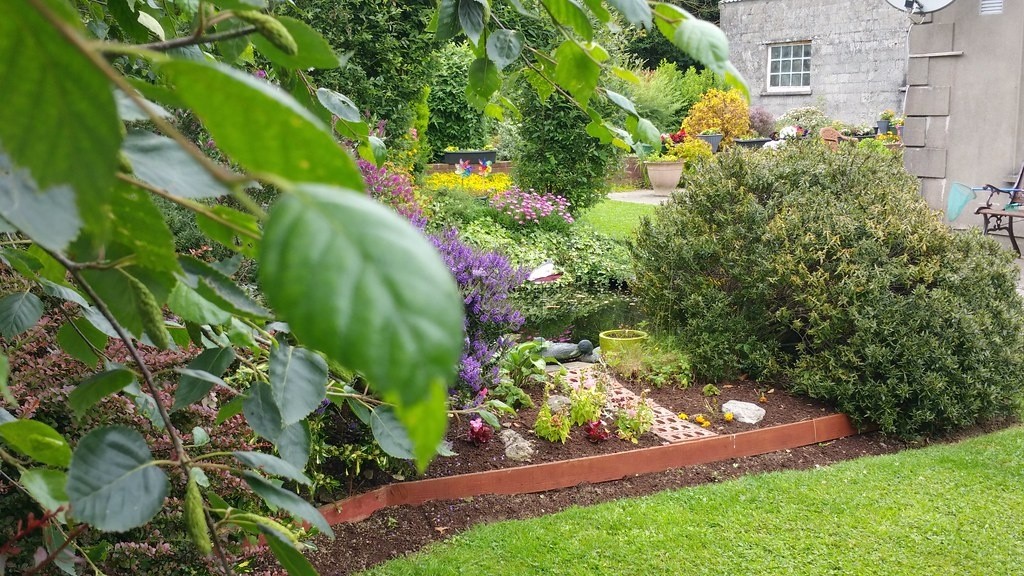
[599,329,648,356]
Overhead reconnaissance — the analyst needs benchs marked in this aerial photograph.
[972,161,1024,258]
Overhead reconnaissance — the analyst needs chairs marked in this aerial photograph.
[819,127,859,152]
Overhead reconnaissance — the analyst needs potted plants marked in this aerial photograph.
[695,127,724,155]
[876,109,895,136]
[635,145,685,192]
[444,144,496,165]
